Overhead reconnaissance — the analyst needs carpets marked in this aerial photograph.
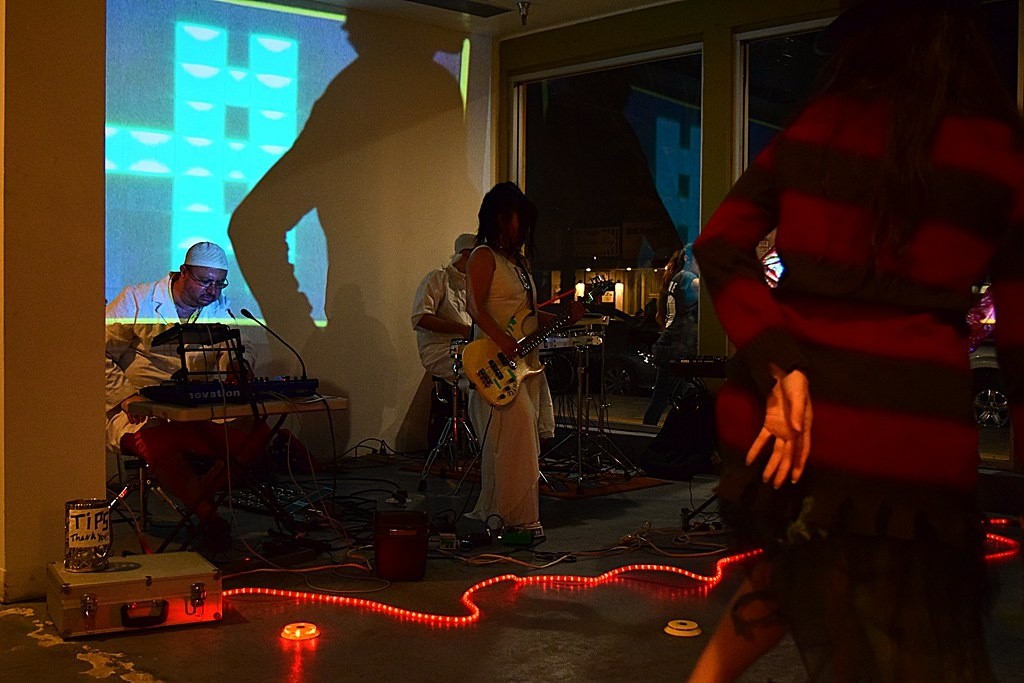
[396,463,674,503]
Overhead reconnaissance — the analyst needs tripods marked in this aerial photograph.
[537,329,646,496]
[416,356,482,492]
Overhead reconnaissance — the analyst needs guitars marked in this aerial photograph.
[462,275,621,407]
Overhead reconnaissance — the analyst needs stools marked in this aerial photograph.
[111,445,182,528]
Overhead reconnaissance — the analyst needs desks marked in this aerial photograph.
[125,393,347,549]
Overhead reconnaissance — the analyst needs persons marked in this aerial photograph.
[684,0,1024,683]
[411,233,555,449]
[642,242,699,426]
[104,241,318,551]
[461,180,587,529]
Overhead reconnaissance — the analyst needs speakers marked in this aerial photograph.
[373,494,429,583]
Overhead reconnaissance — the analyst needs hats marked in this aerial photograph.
[184,241,229,271]
[451,233,479,262]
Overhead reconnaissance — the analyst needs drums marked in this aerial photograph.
[448,337,468,358]
[540,351,574,392]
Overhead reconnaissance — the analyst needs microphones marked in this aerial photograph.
[241,308,308,382]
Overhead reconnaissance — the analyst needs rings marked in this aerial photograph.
[514,349,517,352]
